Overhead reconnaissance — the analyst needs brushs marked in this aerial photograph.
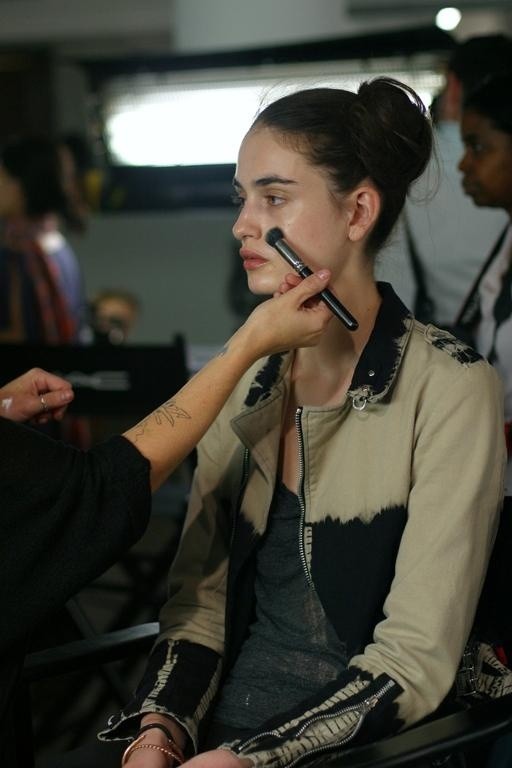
[264,226,361,332]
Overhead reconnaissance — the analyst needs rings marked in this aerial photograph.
[40,394,47,413]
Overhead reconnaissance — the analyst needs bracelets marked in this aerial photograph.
[121,736,185,766]
[134,722,175,744]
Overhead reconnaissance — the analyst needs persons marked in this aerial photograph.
[95,67,506,768]
[372,30,512,341]
[0,128,136,346]
[1,269,333,768]
[449,66,511,496]
